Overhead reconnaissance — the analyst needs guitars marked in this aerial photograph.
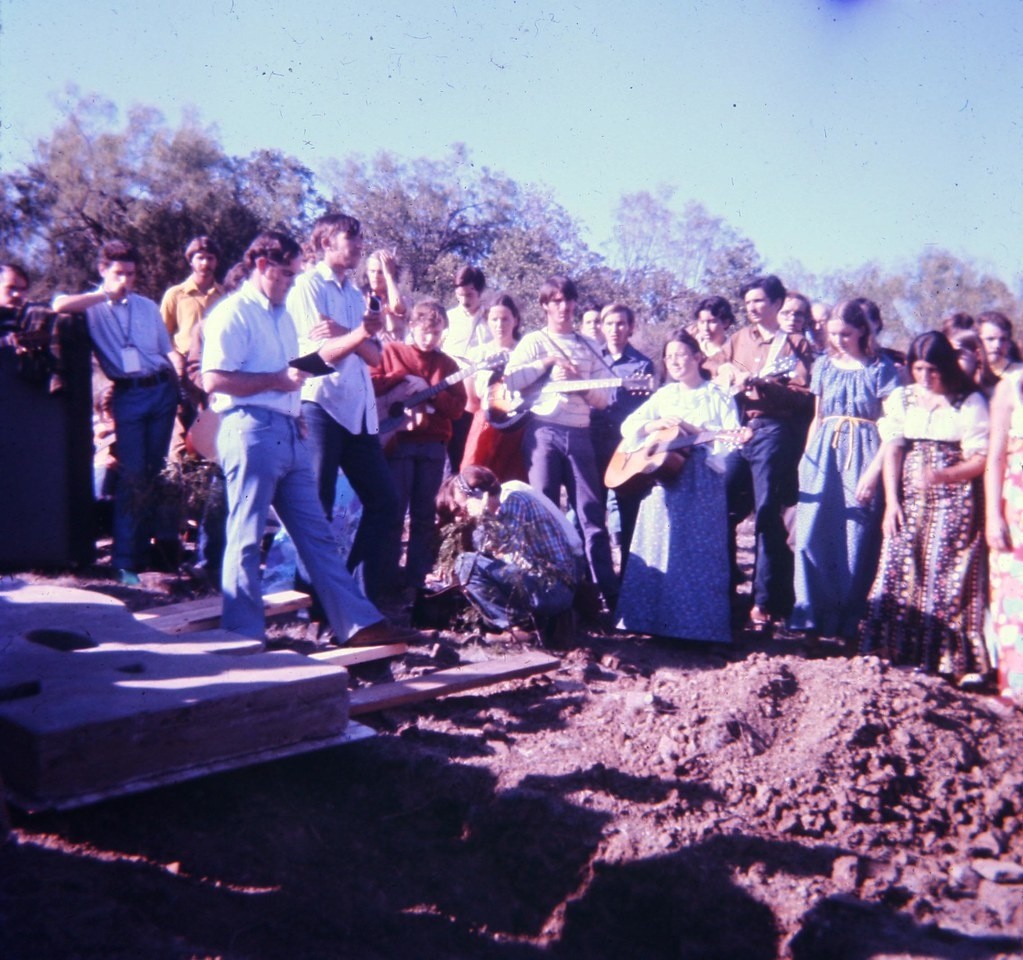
[375,349,511,458]
[712,354,800,424]
[603,423,754,497]
[484,366,656,434]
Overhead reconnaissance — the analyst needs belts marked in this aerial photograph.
[116,368,170,387]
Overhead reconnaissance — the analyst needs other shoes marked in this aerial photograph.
[486,626,540,646]
[742,601,774,635]
[117,568,139,585]
[183,561,209,581]
[349,616,421,641]
[400,577,425,603]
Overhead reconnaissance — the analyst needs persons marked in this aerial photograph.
[943,311,1023,397]
[612,274,908,643]
[159,215,468,650]
[984,365,1023,709]
[52,240,182,590]
[858,331,991,688]
[503,275,619,612]
[449,464,589,643]
[438,264,528,483]
[566,304,655,581]
[0,262,73,368]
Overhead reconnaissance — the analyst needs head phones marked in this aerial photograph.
[456,474,483,500]
